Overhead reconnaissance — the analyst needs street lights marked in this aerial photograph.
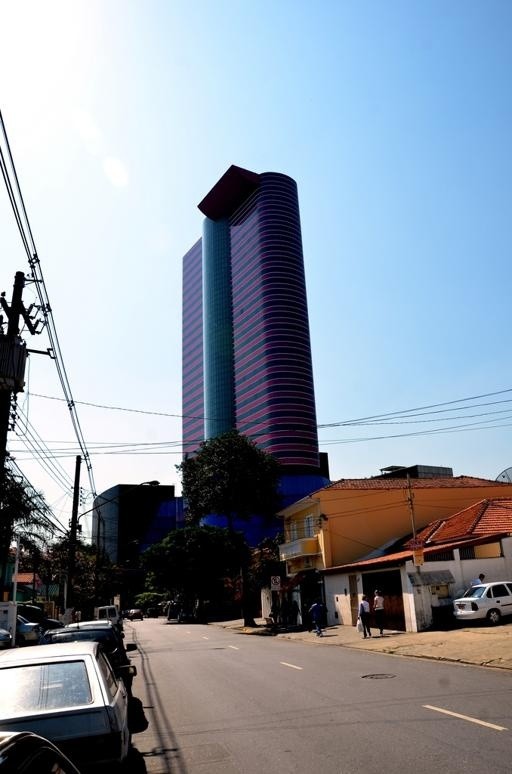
[64,455,161,599]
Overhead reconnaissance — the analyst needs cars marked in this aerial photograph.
[452,581,512,625]
[37,621,137,690]
[0,639,138,769]
[176,605,198,624]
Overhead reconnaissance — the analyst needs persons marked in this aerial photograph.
[357,594,371,639]
[455,573,485,599]
[372,589,385,637]
[270,597,328,637]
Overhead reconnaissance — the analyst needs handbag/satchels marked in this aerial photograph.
[357,616,365,633]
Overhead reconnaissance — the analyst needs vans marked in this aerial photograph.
[94,605,123,631]
[122,608,130,619]
[127,608,144,622]
[1,600,66,651]
